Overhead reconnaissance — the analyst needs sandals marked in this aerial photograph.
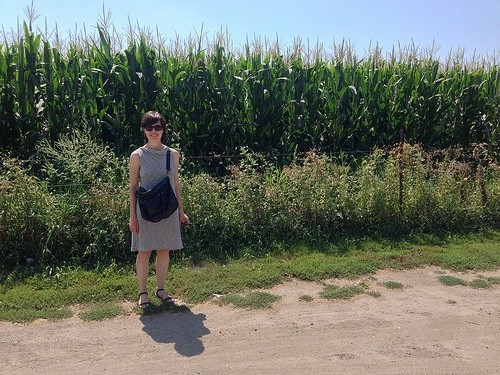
[156,289,174,305]
[138,292,151,310]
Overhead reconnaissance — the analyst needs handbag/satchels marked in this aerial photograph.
[135,176,179,223]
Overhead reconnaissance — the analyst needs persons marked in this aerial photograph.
[129,111,190,309]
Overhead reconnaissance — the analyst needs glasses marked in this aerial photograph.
[144,124,163,131]
